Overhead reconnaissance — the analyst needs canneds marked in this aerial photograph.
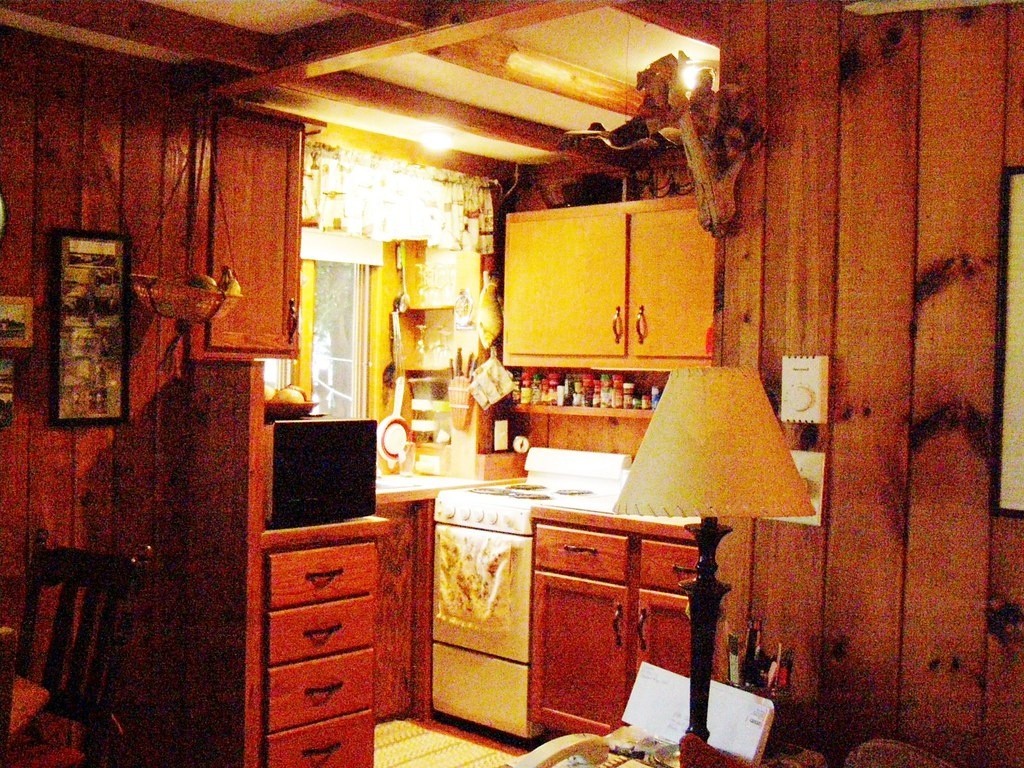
[583,373,635,409]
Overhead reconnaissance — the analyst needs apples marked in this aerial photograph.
[184,273,217,293]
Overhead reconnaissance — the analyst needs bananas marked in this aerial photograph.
[198,264,242,321]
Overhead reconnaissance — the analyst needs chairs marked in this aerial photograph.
[0,527,154,768]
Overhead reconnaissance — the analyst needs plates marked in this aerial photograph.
[265,400,319,419]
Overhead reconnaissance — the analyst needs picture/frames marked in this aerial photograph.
[0,295,34,348]
[45,225,131,428]
[988,165,1024,519]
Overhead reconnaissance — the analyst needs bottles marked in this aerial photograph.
[512,370,661,411]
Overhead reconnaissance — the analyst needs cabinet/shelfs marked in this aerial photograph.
[178,354,388,768]
[502,195,724,371]
[402,304,456,374]
[526,520,698,736]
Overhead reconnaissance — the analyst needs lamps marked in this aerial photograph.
[611,363,816,744]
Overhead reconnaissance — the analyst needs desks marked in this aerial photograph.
[499,725,827,768]
